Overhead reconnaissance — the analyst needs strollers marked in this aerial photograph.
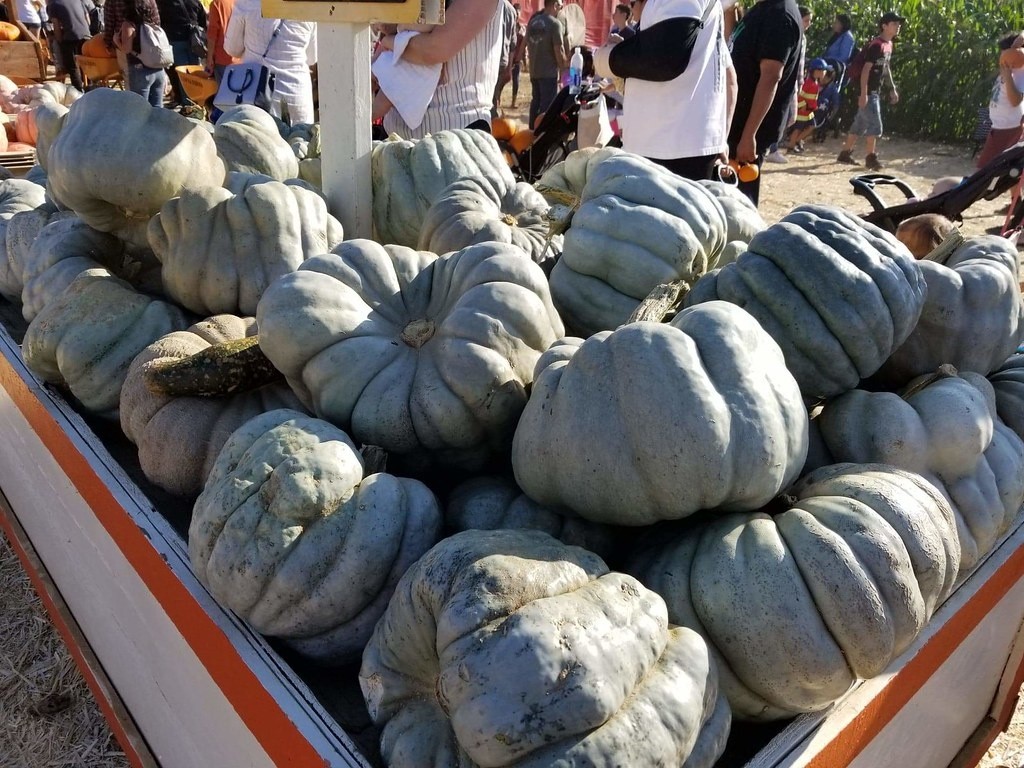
[848,141,1024,238]
[468,76,623,184]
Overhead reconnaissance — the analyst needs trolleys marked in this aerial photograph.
[78,54,125,92]
[174,65,219,106]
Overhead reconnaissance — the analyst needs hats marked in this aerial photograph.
[879,13,906,25]
[808,58,833,71]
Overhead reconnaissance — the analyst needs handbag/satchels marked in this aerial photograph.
[213,61,277,113]
[576,93,615,149]
[972,107,993,145]
[191,26,208,59]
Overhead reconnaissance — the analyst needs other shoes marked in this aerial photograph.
[985,219,1021,239]
[1016,229,1024,247]
[786,138,807,155]
[994,200,1023,216]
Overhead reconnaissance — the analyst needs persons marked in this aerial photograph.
[0,0,902,207]
[976,33,1024,246]
[894,213,956,260]
[905,176,964,204]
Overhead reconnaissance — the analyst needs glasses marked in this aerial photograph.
[517,12,520,16]
[558,4,564,10]
[630,1,639,9]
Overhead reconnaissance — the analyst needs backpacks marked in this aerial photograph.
[847,38,886,81]
[129,21,174,69]
[84,2,105,36]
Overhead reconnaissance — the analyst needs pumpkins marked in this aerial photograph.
[0,21,1024,768]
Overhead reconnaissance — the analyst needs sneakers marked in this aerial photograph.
[836,147,862,166]
[866,152,884,171]
[766,150,790,164]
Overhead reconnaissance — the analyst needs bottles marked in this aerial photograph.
[569,47,584,95]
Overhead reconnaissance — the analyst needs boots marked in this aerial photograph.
[510,94,519,109]
